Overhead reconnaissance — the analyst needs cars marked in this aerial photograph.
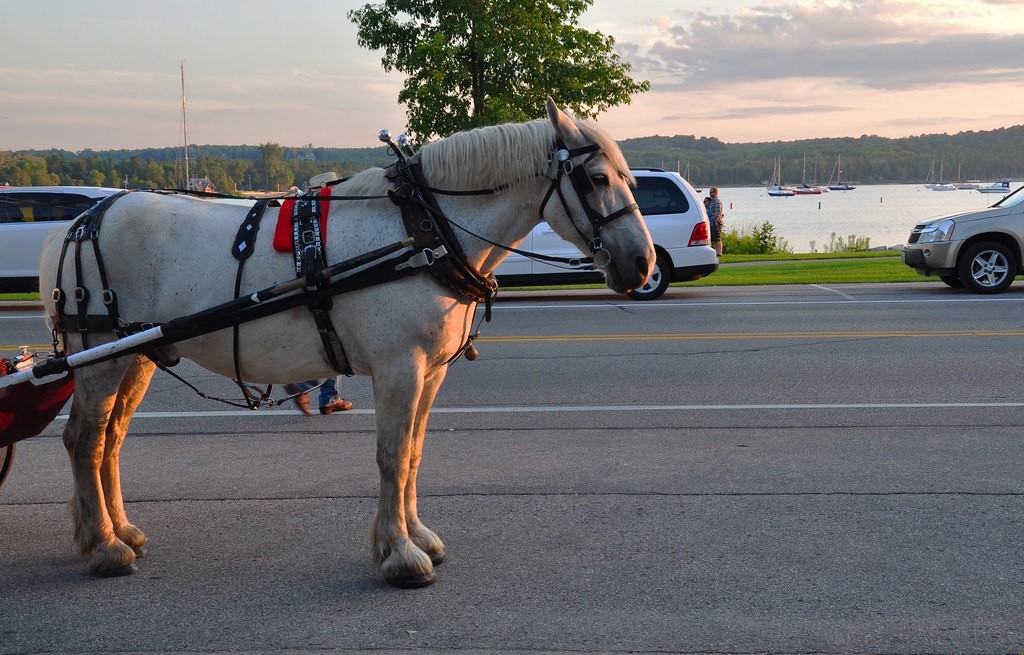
[901,182,1024,295]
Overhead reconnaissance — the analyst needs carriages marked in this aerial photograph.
[0,93,657,588]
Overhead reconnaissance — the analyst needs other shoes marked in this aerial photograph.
[320,400,355,414]
[284,382,314,417]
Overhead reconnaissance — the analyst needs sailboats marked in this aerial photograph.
[677,159,703,193]
[923,158,980,191]
[766,151,856,196]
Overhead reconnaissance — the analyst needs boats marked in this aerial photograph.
[976,179,1011,193]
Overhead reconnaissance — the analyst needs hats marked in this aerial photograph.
[307,173,351,190]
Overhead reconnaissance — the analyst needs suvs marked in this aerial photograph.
[0,183,128,296]
[490,166,720,301]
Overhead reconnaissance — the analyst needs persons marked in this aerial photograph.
[282,171,353,417]
[703,187,724,257]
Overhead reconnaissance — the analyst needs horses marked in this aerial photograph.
[37,95,657,590]
